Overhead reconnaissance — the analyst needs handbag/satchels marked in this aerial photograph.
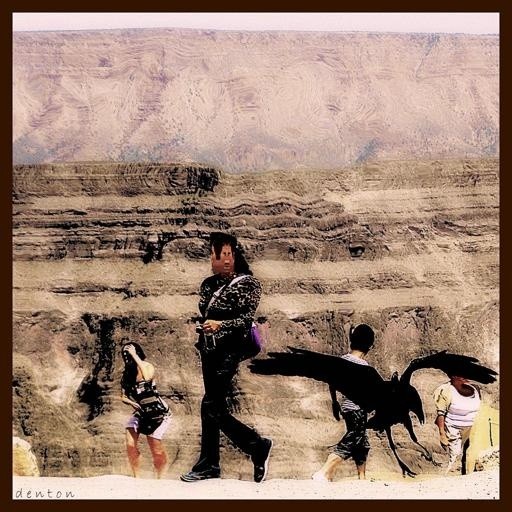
[133,401,166,435]
[239,321,262,363]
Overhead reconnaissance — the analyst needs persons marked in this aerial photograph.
[179,232,274,483]
[312,324,375,482]
[120,342,167,479]
[432,373,481,474]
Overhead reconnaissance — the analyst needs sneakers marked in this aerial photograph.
[312,472,331,482]
[180,466,220,482]
[251,438,273,482]
